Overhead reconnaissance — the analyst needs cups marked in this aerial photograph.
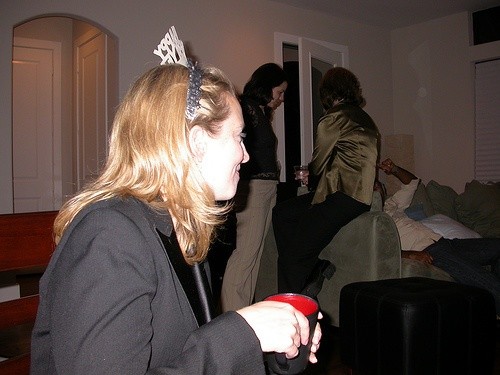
[294,165,308,186]
[263,293,320,375]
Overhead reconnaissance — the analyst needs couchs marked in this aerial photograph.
[312,183,500,375]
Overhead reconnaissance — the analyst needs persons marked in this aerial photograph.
[220,63,287,316]
[277,67,381,300]
[378,158,500,315]
[29,62,323,375]
